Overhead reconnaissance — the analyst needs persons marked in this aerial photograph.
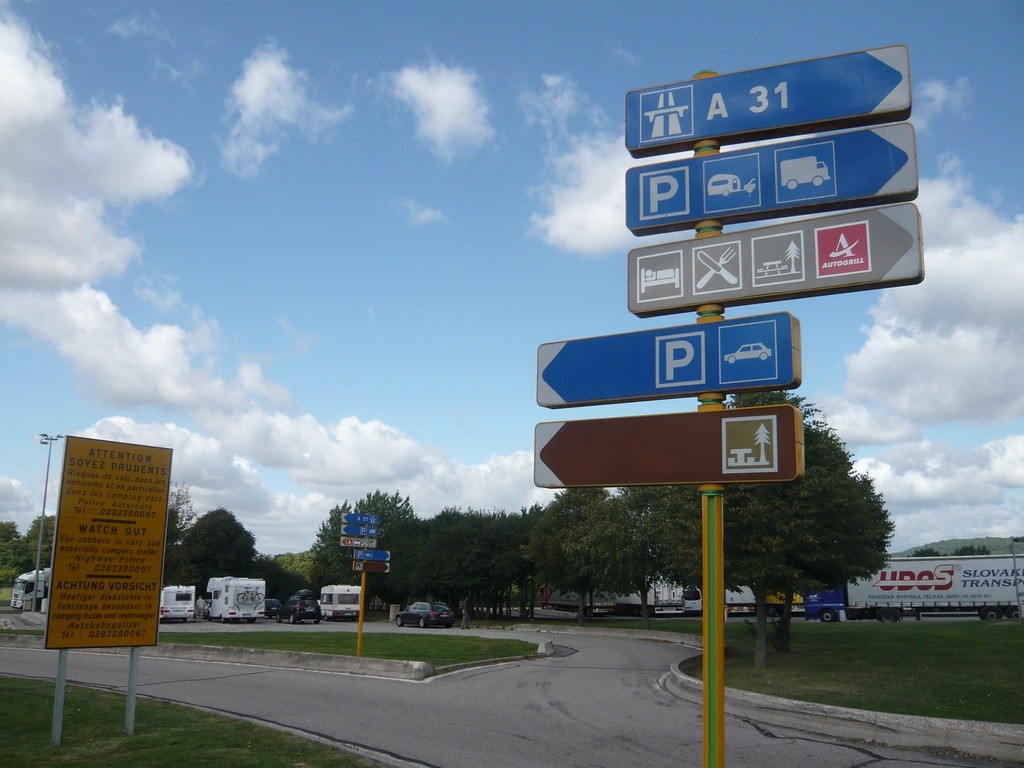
[194,596,206,623]
[461,596,471,629]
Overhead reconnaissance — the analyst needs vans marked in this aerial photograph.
[319,585,361,621]
[159,585,195,623]
[206,577,265,623]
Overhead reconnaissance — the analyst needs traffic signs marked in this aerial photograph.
[342,524,378,537]
[342,536,378,548]
[628,200,925,320]
[621,124,921,239]
[533,405,807,488]
[340,513,379,526]
[353,549,391,561]
[353,561,391,573]
[615,46,915,155]
[535,312,800,409]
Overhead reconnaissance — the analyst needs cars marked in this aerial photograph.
[265,599,281,618]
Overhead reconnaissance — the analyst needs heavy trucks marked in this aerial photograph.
[802,554,1024,624]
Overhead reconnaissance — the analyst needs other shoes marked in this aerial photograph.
[466,627,469,629]
[461,627,464,629]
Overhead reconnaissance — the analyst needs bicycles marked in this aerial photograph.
[236,586,265,605]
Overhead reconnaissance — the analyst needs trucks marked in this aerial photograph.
[682,585,757,617]
[766,589,805,617]
[10,570,44,609]
[546,575,656,617]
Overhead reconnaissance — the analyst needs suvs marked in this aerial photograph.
[395,602,456,628]
[276,589,320,624]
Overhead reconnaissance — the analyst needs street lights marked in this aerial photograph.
[30,433,65,613]
[1010,536,1023,619]
